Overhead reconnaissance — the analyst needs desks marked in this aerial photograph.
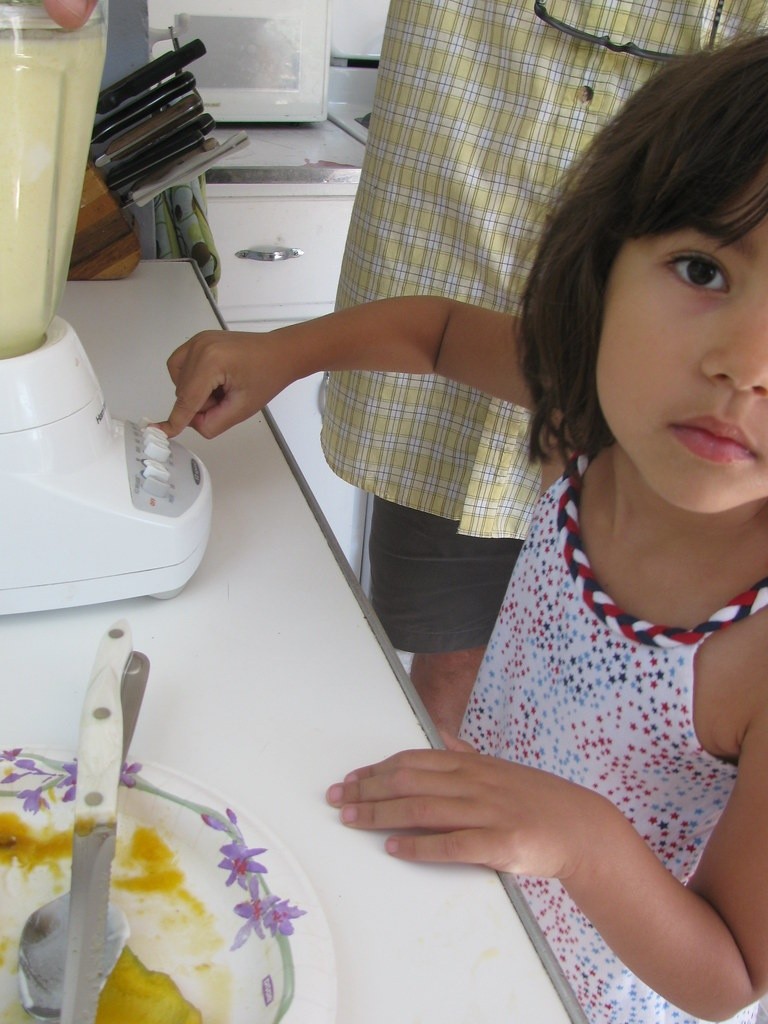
[0,251,588,1024]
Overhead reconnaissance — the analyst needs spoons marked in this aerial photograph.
[17,650,153,1024]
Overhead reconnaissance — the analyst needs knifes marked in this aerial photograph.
[58,618,133,1024]
[88,39,251,212]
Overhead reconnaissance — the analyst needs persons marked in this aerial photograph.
[146,29,768,1023]
[40,0,768,738]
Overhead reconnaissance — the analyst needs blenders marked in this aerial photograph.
[0,2,214,618]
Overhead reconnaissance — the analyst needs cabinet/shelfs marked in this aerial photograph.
[205,195,368,584]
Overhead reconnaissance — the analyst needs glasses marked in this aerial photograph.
[533,0,725,64]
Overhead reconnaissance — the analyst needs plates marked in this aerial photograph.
[0,739,340,1024]
